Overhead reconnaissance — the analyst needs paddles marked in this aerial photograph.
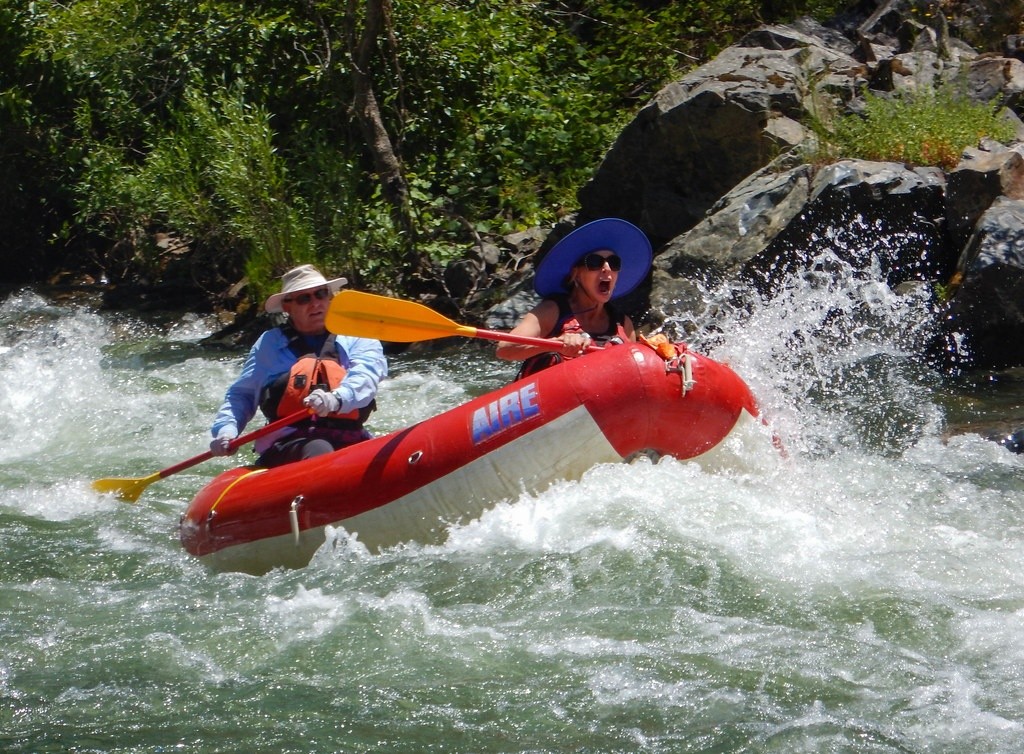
[324,290,604,354]
[90,403,318,504]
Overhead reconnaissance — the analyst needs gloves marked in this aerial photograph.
[209,425,239,457]
[304,389,340,417]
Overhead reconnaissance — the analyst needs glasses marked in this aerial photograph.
[572,253,622,272]
[281,288,330,305]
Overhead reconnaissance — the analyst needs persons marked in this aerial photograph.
[210,263,387,470]
[495,217,673,361]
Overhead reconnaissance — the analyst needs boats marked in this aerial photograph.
[178,341,789,583]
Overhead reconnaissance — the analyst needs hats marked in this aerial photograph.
[533,218,653,300]
[264,264,348,313]
